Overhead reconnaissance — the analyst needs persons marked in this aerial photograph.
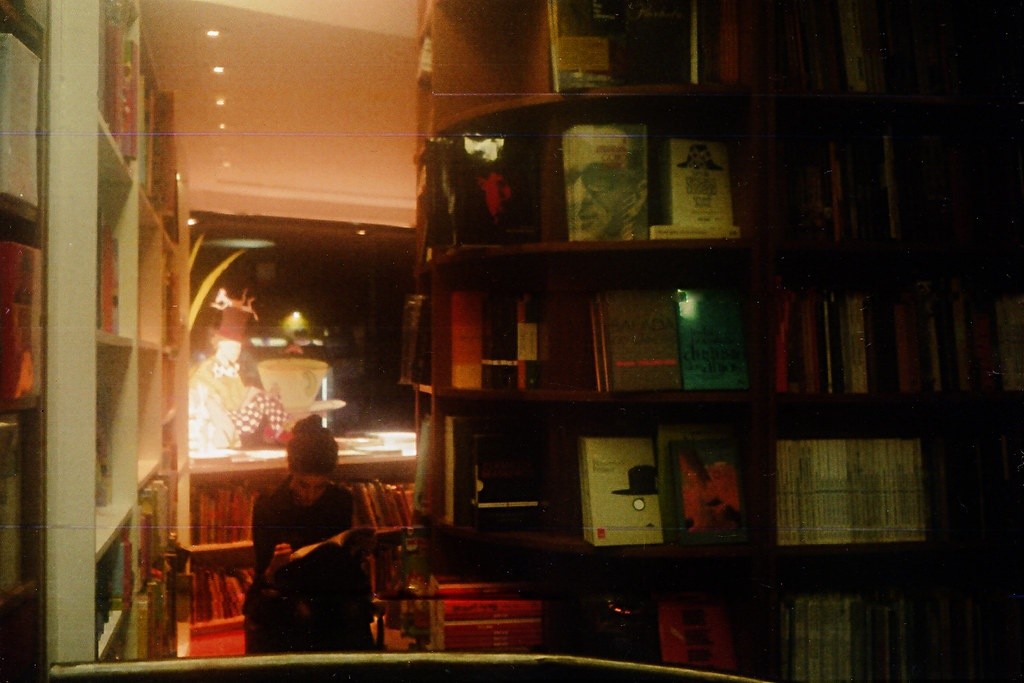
[241,415,380,654]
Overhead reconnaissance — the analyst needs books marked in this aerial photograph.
[415,113,1024,249]
[191,562,254,624]
[439,573,1024,683]
[413,415,1024,548]
[103,0,138,163]
[188,482,278,544]
[341,478,416,530]
[544,0,1024,97]
[402,280,1023,394]
[0,217,183,597]
[145,85,181,244]
[290,526,376,558]
[94,479,179,661]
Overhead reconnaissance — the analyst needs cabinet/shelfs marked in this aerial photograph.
[0,0,1024,683]
